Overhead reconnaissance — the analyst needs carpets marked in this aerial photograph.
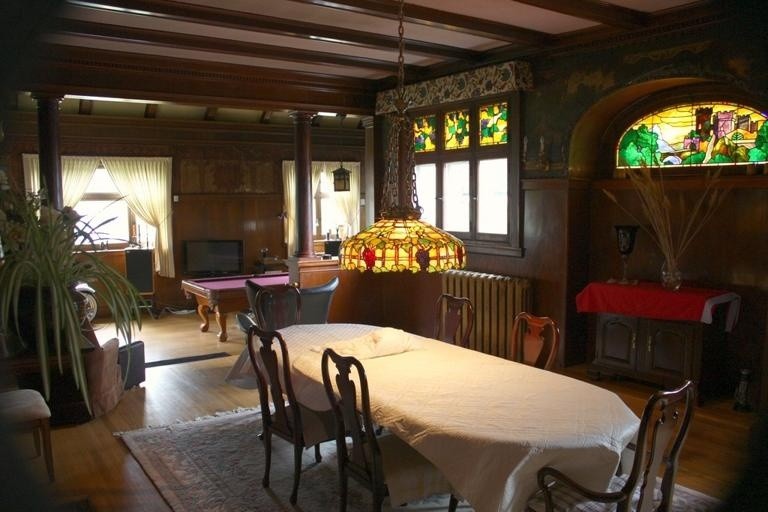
[114,396,731,510]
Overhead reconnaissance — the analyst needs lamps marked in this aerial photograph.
[331,112,352,191]
[338,0,468,279]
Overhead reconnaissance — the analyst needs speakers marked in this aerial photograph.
[124,247,156,295]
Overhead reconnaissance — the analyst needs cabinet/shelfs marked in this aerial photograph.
[590,279,730,406]
[126,290,156,320]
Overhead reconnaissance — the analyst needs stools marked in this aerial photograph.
[0,389,58,484]
[116,339,146,392]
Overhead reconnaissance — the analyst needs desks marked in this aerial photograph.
[267,323,644,511]
[1,334,96,428]
[181,271,290,342]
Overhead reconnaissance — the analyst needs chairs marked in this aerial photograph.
[72,291,125,418]
[320,348,461,511]
[245,325,364,507]
[225,273,340,381]
[508,311,561,370]
[431,294,475,348]
[251,282,304,347]
[528,378,698,512]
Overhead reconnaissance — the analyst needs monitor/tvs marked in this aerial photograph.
[324,241,341,255]
[182,239,244,276]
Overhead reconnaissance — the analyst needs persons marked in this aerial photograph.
[0,200,25,255]
[683,107,712,151]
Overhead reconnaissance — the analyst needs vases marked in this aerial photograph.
[660,265,681,291]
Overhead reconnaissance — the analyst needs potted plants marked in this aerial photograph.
[1,172,158,417]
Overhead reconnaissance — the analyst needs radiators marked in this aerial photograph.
[441,268,535,362]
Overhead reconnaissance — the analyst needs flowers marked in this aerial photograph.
[1,178,83,254]
